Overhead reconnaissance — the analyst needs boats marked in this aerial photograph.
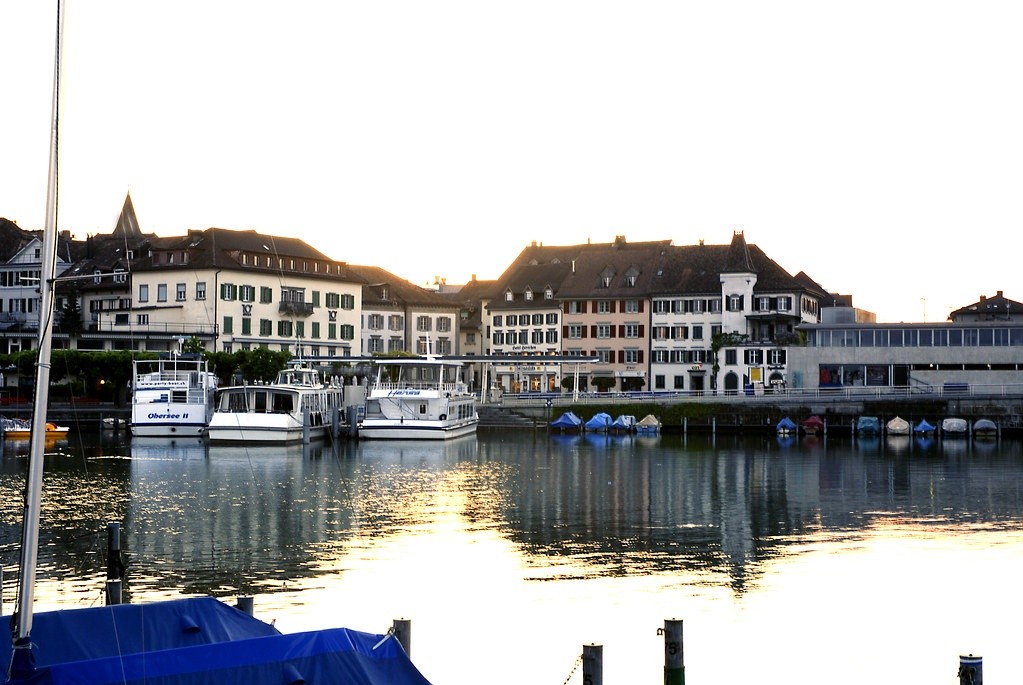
[941,419,969,436]
[550,411,582,434]
[204,332,345,446]
[857,416,880,433]
[914,417,935,435]
[0,0,432,684]
[609,415,631,436]
[975,419,998,436]
[357,360,481,441]
[4,421,70,438]
[585,412,614,433]
[802,416,823,435]
[777,417,795,434]
[101,417,126,430]
[635,415,662,434]
[128,334,219,438]
[885,417,909,436]
